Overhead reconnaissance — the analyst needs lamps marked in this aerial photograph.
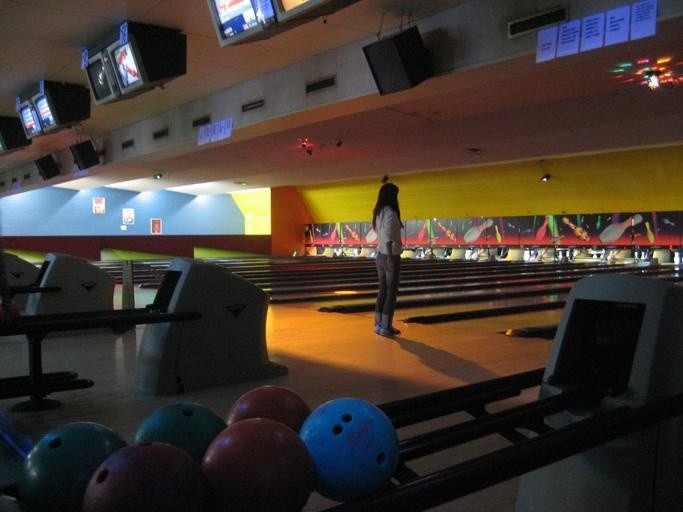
[153,171,162,180]
[540,175,551,182]
[646,71,662,88]
[301,139,313,156]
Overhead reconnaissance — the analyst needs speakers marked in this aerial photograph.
[362,26,432,97]
[69,140,99,170]
[34,154,61,181]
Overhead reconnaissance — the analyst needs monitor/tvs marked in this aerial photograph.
[31,81,90,133]
[206,0,319,47]
[105,35,186,94]
[272,0,357,24]
[0,116,32,155]
[16,101,43,140]
[83,51,155,106]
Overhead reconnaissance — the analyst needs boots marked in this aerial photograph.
[372,312,401,337]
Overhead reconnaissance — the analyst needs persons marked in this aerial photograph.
[370,182,406,338]
